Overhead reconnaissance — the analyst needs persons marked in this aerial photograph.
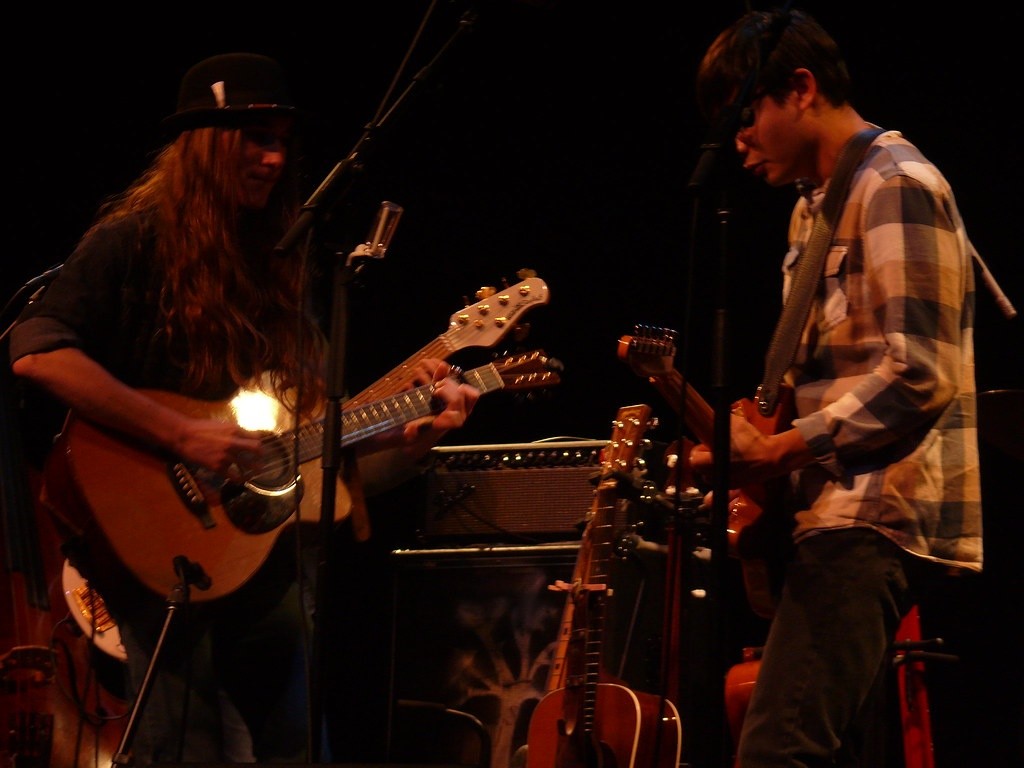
[7,53,481,767]
[695,11,982,768]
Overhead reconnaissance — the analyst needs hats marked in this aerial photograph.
[159,53,295,127]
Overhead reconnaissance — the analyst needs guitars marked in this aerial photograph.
[616,324,827,623]
[61,273,553,701]
[47,353,562,620]
[522,402,681,765]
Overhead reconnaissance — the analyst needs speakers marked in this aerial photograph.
[383,540,666,768]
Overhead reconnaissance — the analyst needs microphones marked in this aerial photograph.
[689,136,739,206]
